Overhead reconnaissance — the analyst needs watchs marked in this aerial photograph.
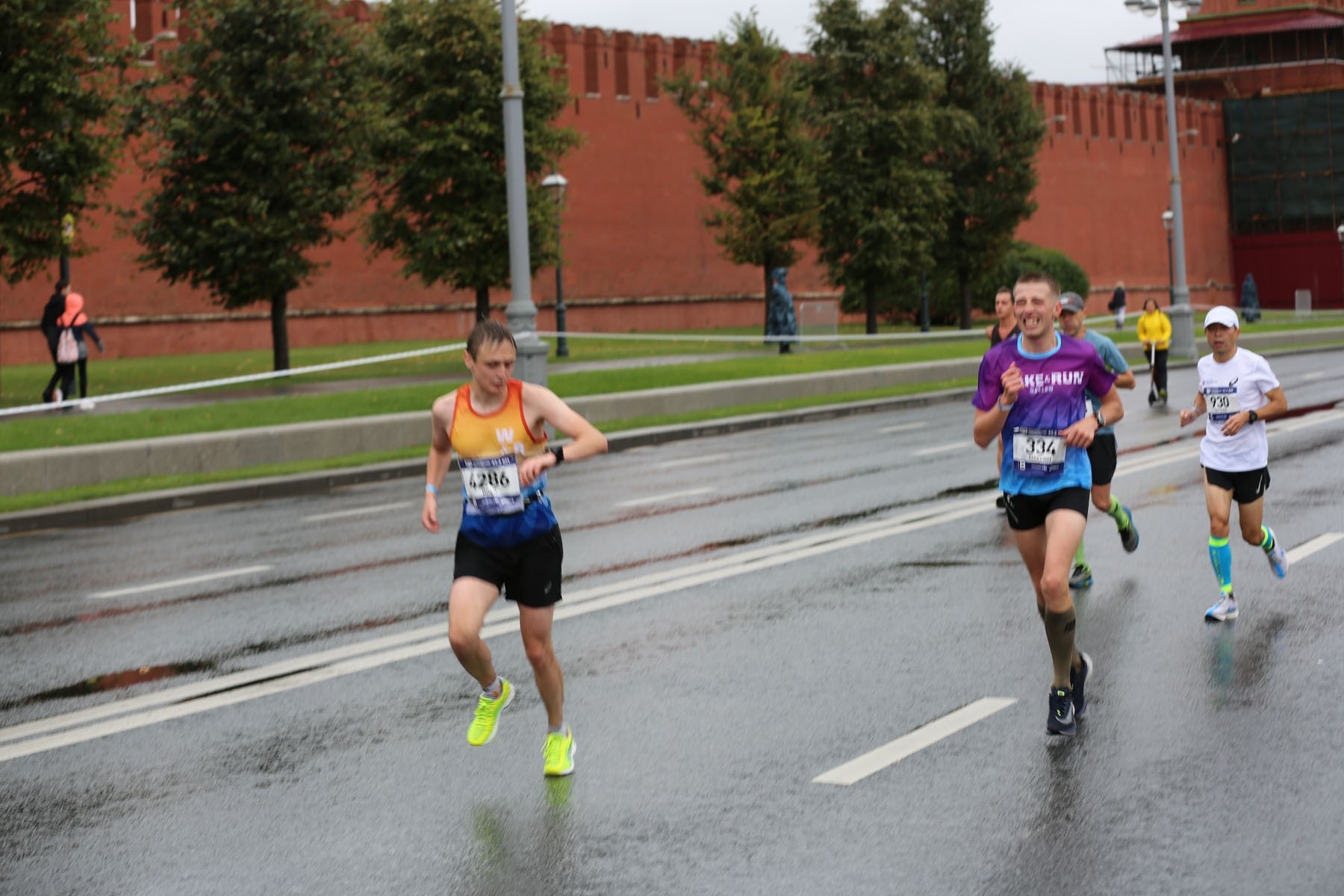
[1092,411,1106,431]
[1248,410,1257,424]
[543,445,562,468]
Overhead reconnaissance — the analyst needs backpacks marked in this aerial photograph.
[56,312,82,363]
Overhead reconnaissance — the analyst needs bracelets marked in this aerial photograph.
[1191,407,1201,417]
[425,484,438,498]
[997,395,1013,412]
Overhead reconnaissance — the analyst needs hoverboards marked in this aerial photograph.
[1143,338,1171,408]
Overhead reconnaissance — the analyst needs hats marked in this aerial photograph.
[1059,292,1084,313]
[1203,305,1240,330]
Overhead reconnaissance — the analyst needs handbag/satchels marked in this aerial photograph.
[1108,301,1115,311]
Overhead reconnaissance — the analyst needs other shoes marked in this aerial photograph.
[996,495,1007,514]
[80,398,95,410]
[54,389,62,407]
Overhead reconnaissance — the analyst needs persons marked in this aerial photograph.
[40,283,105,412]
[971,270,1290,737]
[422,320,608,778]
[769,270,799,353]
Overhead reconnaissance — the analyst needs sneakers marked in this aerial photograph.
[1046,685,1077,735]
[1204,592,1239,622]
[1118,506,1139,552]
[1070,651,1093,720]
[540,723,577,775]
[1265,526,1289,579]
[467,676,515,746]
[1069,565,1093,588]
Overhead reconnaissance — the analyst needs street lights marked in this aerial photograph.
[540,171,571,359]
[1122,0,1203,360]
